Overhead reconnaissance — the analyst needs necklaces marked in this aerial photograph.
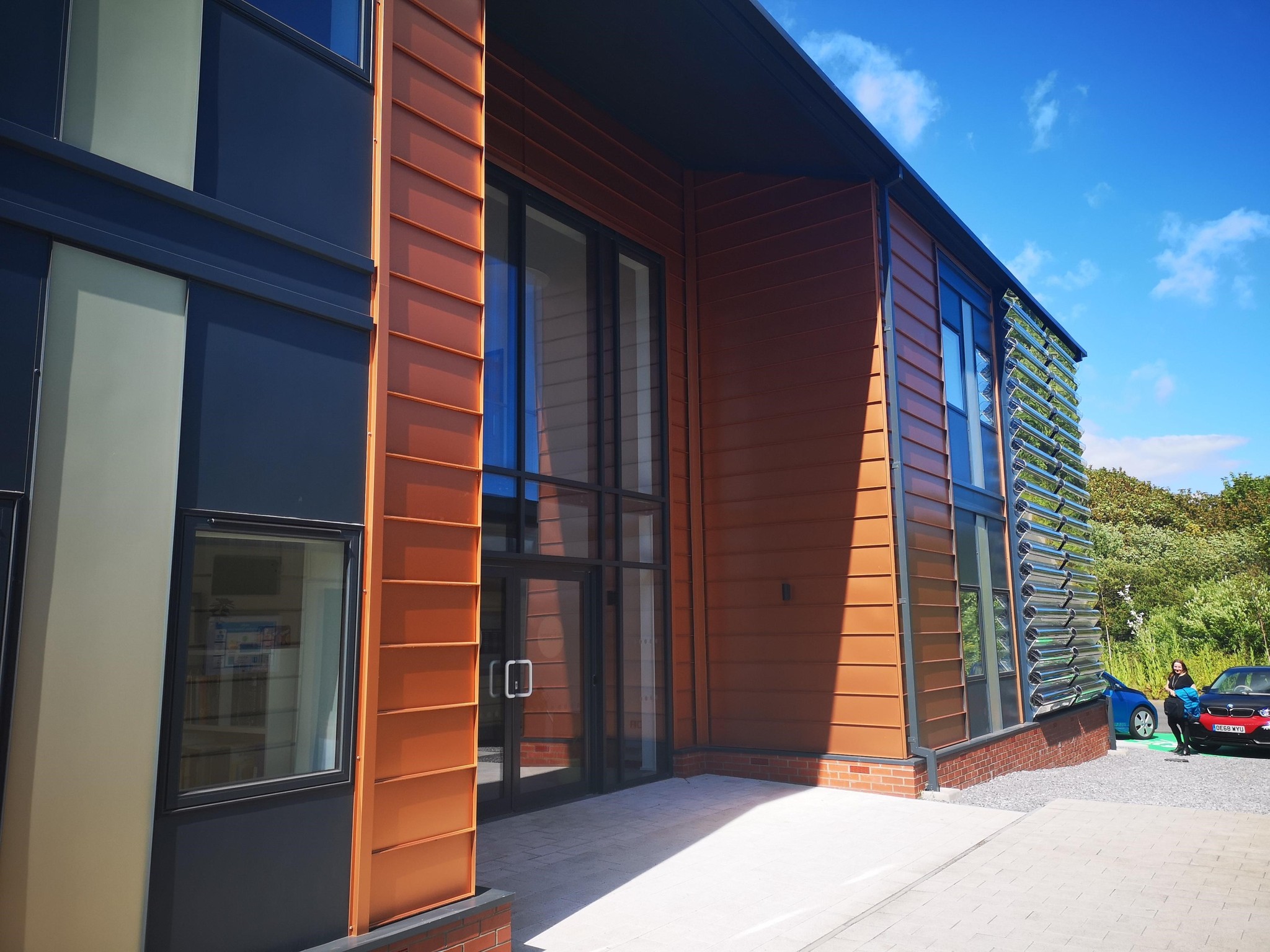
[1172,675,1180,690]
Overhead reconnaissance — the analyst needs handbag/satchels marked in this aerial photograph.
[1164,696,1184,717]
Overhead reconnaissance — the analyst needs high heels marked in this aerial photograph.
[1179,748,1190,756]
[1172,742,1184,753]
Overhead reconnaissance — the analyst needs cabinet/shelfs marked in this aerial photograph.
[183,645,300,778]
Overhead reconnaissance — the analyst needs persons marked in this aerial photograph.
[1165,659,1198,756]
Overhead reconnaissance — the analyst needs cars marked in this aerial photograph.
[970,653,1158,740]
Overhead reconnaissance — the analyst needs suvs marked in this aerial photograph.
[1188,666,1270,753]
